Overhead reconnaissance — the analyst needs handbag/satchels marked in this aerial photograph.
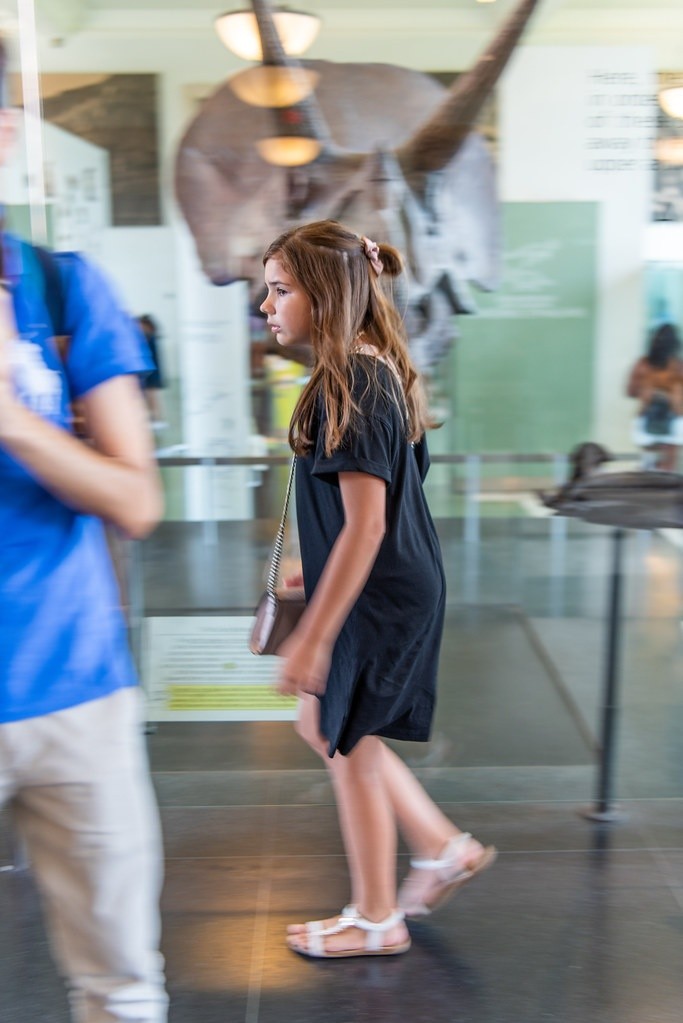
[247,589,308,658]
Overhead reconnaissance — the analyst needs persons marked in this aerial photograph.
[627,322,683,450]
[1,40,172,1022]
[259,214,496,958]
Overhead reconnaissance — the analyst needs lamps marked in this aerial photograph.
[252,108,324,167]
[213,0,323,60]
[228,64,322,109]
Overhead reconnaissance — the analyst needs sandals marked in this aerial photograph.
[283,905,412,958]
[395,832,499,922]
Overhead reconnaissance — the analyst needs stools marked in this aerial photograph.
[531,469,683,824]
